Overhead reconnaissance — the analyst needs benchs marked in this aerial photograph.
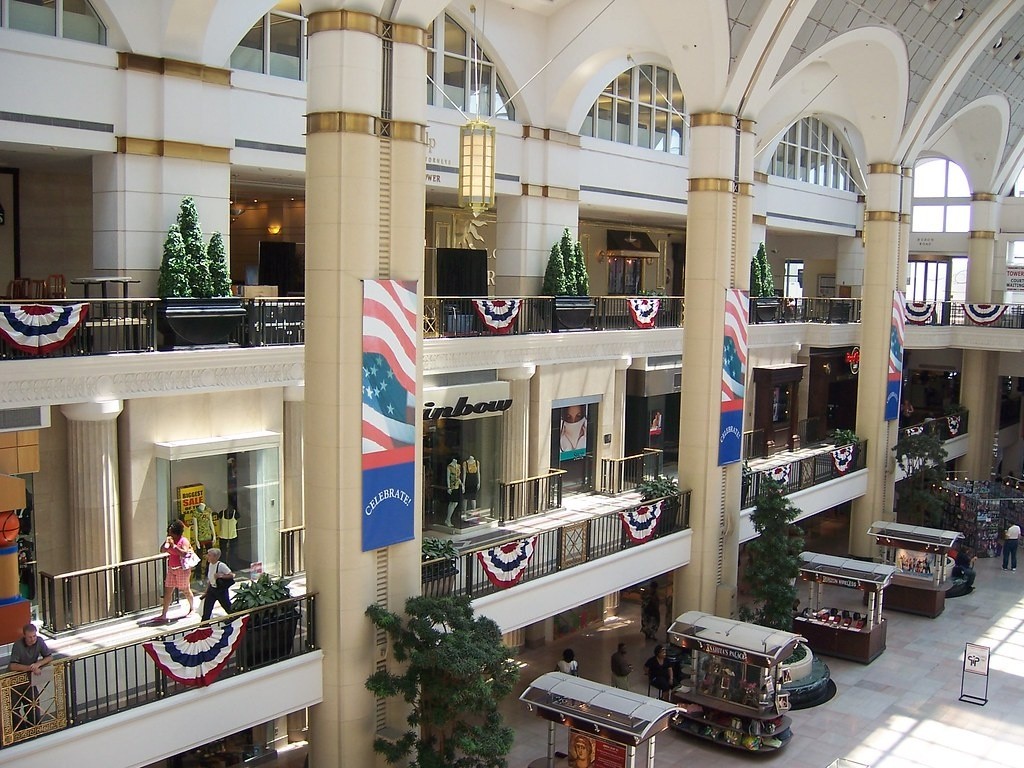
[944,576,973,598]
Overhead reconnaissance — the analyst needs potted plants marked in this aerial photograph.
[751,241,783,324]
[830,429,861,471]
[530,226,596,331]
[742,460,753,508]
[421,536,460,600]
[142,194,248,350]
[230,571,302,668]
[634,475,680,535]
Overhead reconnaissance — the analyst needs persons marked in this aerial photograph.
[560,404,587,452]
[639,581,660,642]
[1008,470,1015,479]
[200,549,238,628]
[643,643,675,701]
[445,458,465,528]
[609,642,632,690]
[954,545,977,589]
[901,398,914,428]
[6,623,55,731]
[652,411,662,429]
[150,520,195,623]
[772,390,778,420]
[174,502,241,587]
[463,455,481,511]
[554,648,579,677]
[791,599,806,618]
[1000,519,1021,571]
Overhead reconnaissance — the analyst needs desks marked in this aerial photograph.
[70,276,141,353]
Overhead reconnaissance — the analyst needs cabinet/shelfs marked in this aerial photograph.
[668,690,795,754]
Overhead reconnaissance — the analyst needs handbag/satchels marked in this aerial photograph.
[216,561,235,591]
[180,538,201,570]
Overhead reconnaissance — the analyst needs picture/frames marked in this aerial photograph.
[777,693,789,710]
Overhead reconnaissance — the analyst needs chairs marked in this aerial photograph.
[8,278,46,298]
[46,273,68,299]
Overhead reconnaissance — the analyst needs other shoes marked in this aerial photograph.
[1002,566,1007,570]
[1010,566,1016,570]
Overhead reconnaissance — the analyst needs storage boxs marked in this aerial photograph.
[232,285,279,302]
[853,612,861,621]
[842,610,849,619]
[843,617,853,627]
[830,608,838,616]
[821,613,829,622]
[856,620,864,629]
[832,615,841,624]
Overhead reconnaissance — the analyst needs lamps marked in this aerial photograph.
[875,536,880,541]
[886,538,891,543]
[766,658,772,665]
[527,703,534,712]
[857,581,862,587]
[934,545,940,551]
[593,723,600,732]
[673,634,681,642]
[633,735,641,743]
[697,640,704,649]
[822,361,832,376]
[671,709,680,721]
[559,713,567,722]
[925,544,931,550]
[230,174,246,216]
[815,574,819,580]
[267,226,281,234]
